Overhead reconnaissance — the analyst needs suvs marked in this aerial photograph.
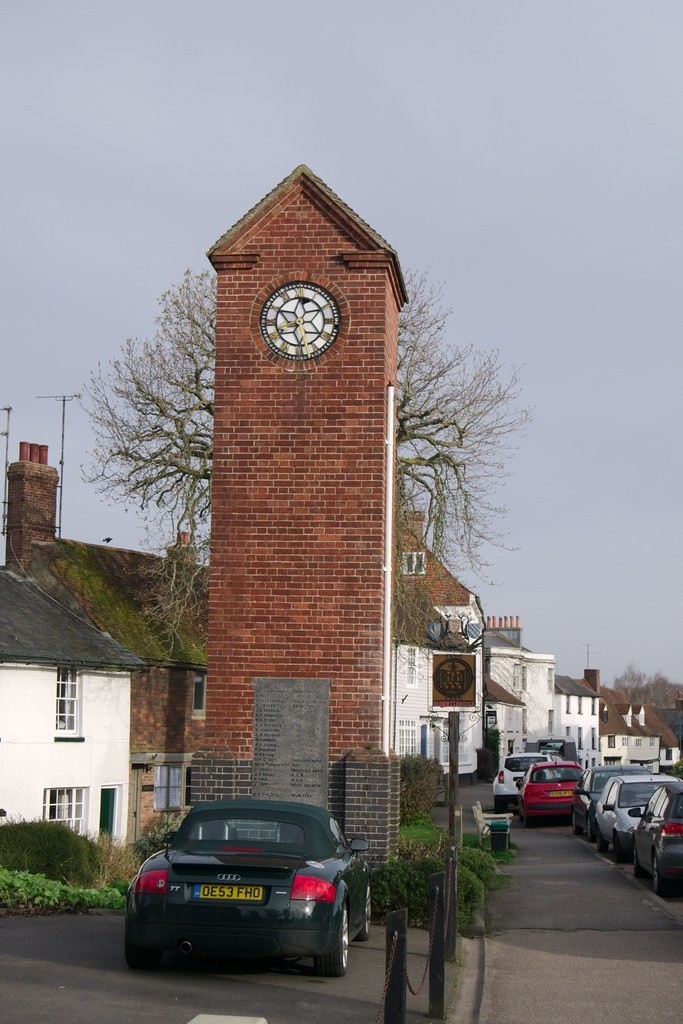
[492,751,566,814]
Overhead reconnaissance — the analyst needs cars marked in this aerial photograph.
[628,782,683,898]
[124,800,372,977]
[571,765,652,843]
[515,760,586,828]
[594,773,683,864]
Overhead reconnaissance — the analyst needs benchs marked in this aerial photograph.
[472,801,514,846]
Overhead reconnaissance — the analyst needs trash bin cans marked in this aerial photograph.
[485,820,511,852]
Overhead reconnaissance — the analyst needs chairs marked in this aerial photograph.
[276,821,304,843]
[202,821,228,840]
[561,770,577,779]
[535,770,547,781]
[622,790,636,803]
[594,777,606,789]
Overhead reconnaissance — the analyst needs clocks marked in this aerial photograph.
[248,272,351,372]
